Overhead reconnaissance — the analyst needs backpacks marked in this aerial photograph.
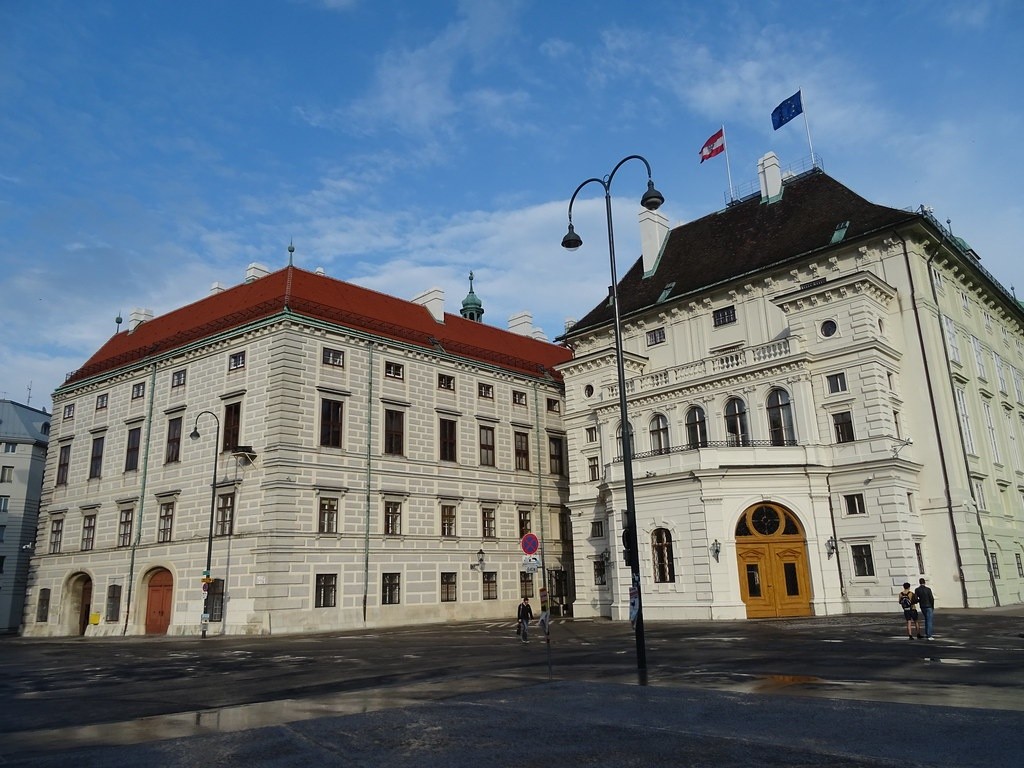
[901,591,911,608]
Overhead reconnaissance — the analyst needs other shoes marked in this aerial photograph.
[909,635,915,640]
[917,633,924,639]
[522,639,531,643]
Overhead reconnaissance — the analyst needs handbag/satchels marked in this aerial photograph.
[516,624,521,635]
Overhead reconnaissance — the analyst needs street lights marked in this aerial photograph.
[188,409,222,636]
[562,153,667,687]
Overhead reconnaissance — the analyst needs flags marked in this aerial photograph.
[771,92,804,131]
[698,129,727,166]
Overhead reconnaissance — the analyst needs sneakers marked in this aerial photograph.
[928,637,934,641]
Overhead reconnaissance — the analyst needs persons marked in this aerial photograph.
[518,598,533,643]
[898,583,924,641]
[915,579,937,640]
